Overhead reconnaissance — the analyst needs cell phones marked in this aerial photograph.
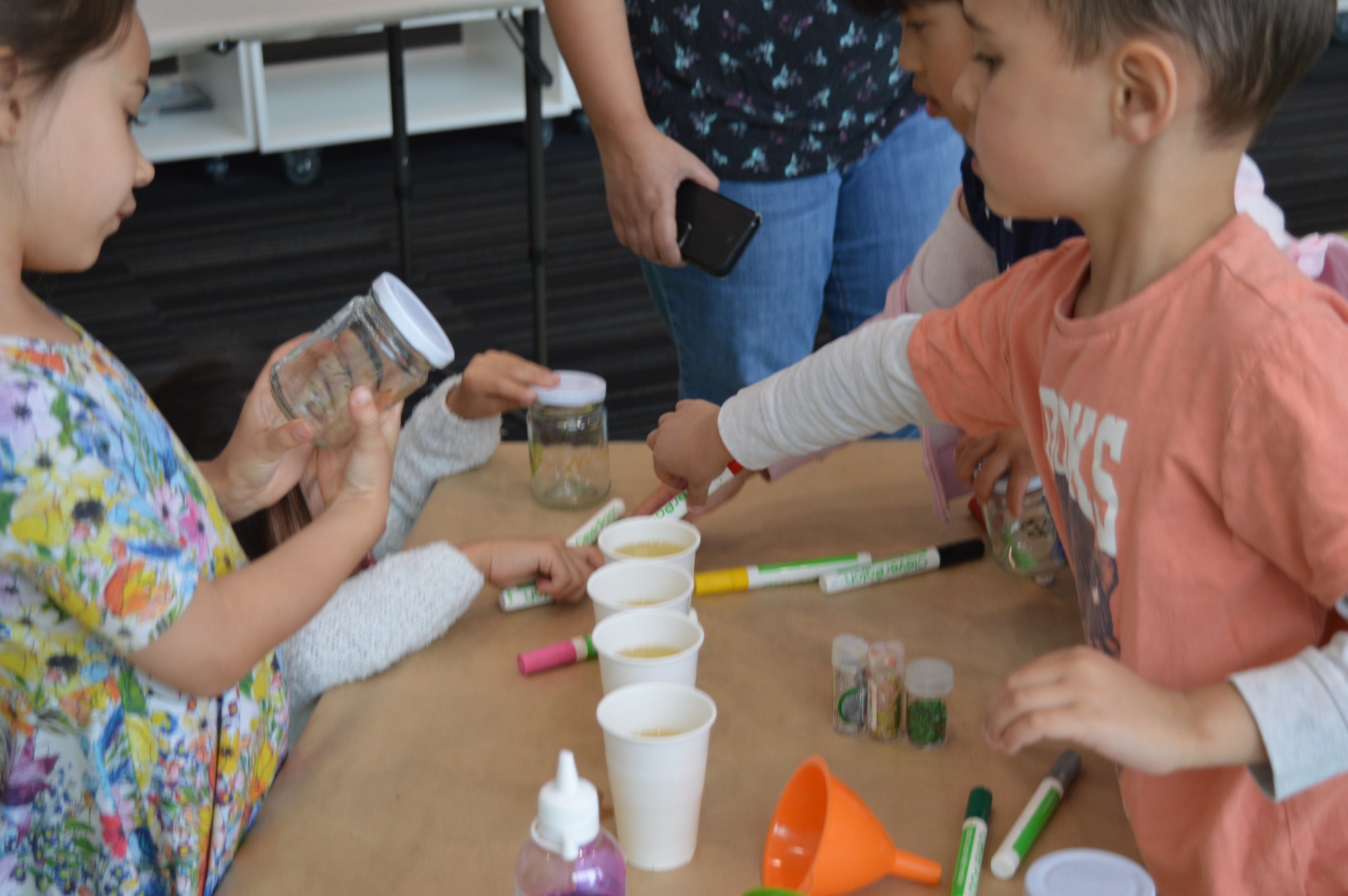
[674,178,762,276]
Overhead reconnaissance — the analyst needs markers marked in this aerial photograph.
[695,552,872,596]
[818,538,986,596]
[517,633,600,677]
[499,581,553,612]
[652,460,741,519]
[565,498,624,549]
[989,749,1083,879]
[950,785,993,896]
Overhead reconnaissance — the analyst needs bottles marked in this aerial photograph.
[270,272,455,450]
[905,659,953,748]
[528,370,611,509]
[831,634,869,735]
[516,750,627,896]
[972,467,1067,577]
[867,643,903,740]
[1023,849,1156,896]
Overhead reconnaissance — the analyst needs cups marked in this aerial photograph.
[586,559,695,626]
[596,683,718,871]
[599,517,701,578]
[592,609,704,697]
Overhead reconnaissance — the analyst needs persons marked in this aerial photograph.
[542,0,966,400]
[0,2,402,896]
[142,351,604,757]
[650,0,1348,896]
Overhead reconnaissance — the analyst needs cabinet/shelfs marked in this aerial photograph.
[130,7,584,190]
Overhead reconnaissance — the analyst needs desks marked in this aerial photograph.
[212,439,1139,896]
[131,0,554,368]
